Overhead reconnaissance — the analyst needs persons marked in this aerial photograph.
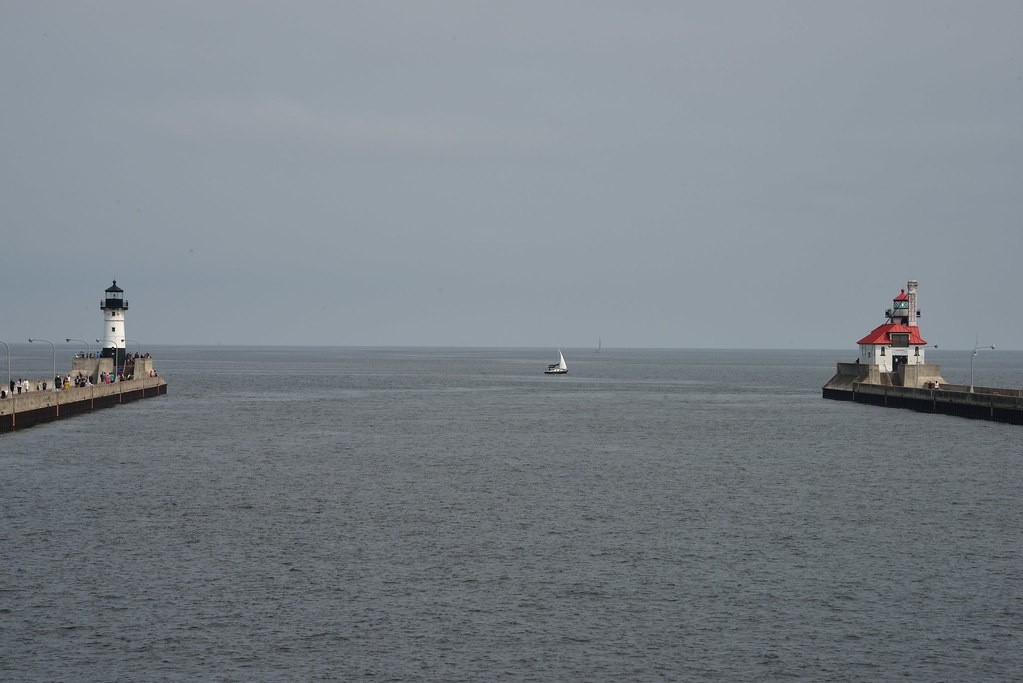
[928,381,933,390]
[0,350,158,394]
[934,381,939,390]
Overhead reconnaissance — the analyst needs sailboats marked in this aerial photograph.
[542,349,569,376]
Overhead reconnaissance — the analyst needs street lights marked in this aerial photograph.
[96,338,118,382]
[120,339,142,380]
[29,338,56,391]
[916,344,939,388]
[66,338,89,385]
[970,344,996,394]
